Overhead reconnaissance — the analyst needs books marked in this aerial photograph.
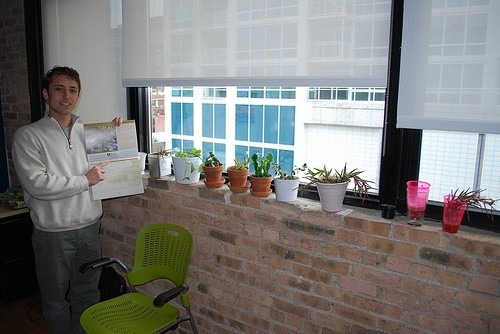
[83,119,144,200]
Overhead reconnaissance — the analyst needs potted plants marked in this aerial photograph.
[271,162,308,202]
[292,162,381,213]
[170,147,203,184]
[148,149,172,177]
[226,155,250,193]
[249,153,273,196]
[202,152,226,188]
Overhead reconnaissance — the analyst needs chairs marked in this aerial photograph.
[78,223,199,334]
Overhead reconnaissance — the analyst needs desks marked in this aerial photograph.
[1,197,30,220]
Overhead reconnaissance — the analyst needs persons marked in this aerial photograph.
[12,66,122,334]
